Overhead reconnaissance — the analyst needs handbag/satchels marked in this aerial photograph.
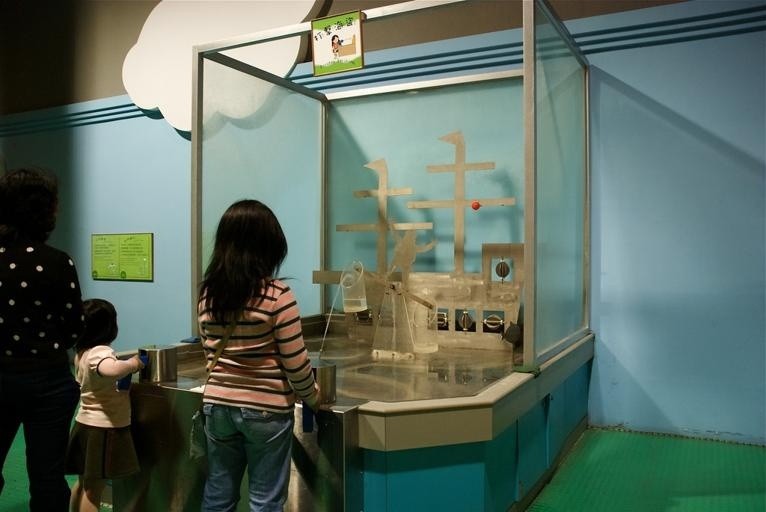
[187,410,207,462]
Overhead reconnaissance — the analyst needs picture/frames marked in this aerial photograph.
[310,9,364,77]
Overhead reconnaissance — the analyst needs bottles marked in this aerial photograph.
[414,287,439,355]
[341,268,369,314]
[372,282,415,360]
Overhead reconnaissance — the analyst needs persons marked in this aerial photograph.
[63,298,145,512]
[0,169,85,512]
[197,199,321,512]
